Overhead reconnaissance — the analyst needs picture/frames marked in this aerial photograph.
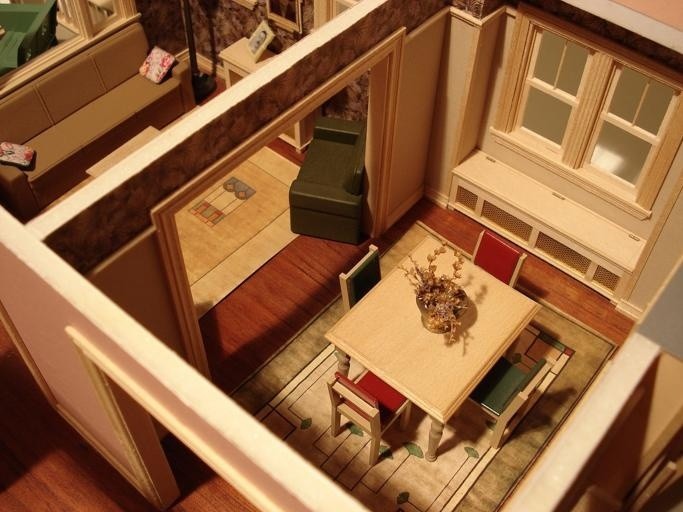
[247,0,304,62]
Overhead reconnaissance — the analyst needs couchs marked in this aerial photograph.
[288,117,367,247]
[0,22,194,221]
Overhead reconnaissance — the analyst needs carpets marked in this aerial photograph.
[226,218,618,512]
[169,141,304,328]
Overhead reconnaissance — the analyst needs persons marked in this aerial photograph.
[250,30,266,54]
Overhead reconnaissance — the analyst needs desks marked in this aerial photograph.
[214,36,314,152]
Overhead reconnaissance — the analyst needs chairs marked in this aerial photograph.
[3,0,57,72]
[340,244,381,312]
[326,368,412,467]
[472,229,528,287]
[468,355,553,449]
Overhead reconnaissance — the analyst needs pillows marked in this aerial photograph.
[137,45,175,83]
[1,139,35,171]
